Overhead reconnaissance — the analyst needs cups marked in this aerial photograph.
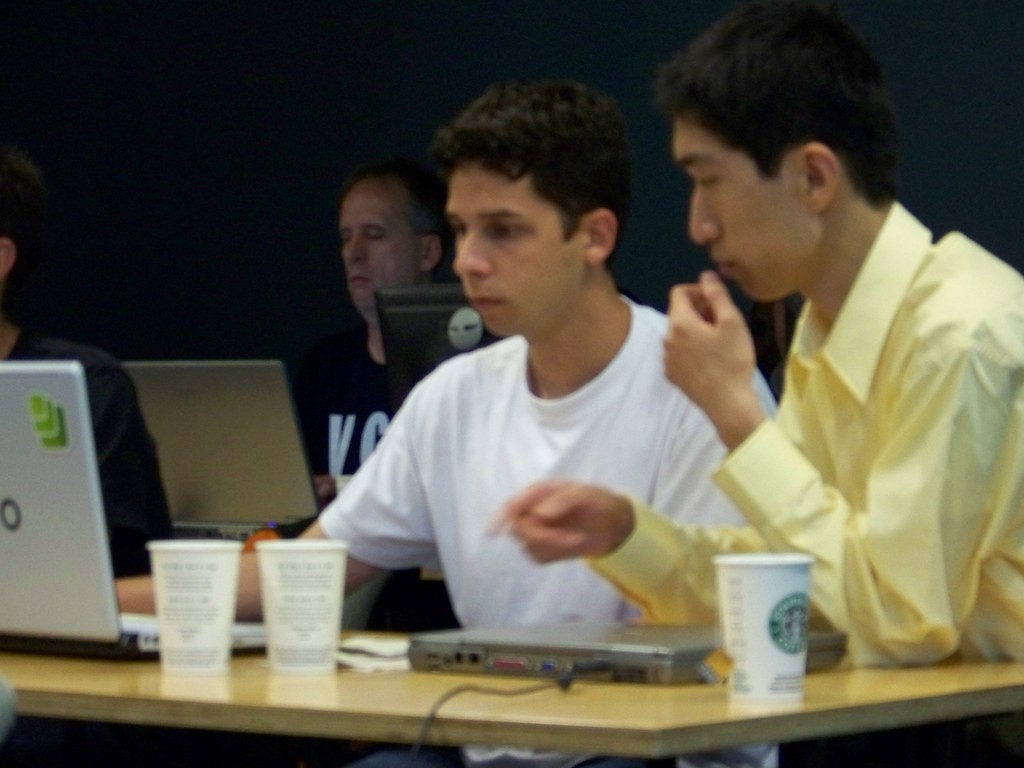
[255,538,348,674]
[712,552,813,713]
[147,539,245,677]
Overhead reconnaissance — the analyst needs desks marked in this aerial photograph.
[1,628,1024,761]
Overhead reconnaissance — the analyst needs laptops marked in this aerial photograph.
[373,280,508,428]
[408,620,849,685]
[125,360,324,543]
[0,360,271,662]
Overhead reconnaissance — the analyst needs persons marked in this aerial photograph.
[487,1,1024,768]
[112,72,782,768]
[0,193,172,581]
[293,161,460,505]
